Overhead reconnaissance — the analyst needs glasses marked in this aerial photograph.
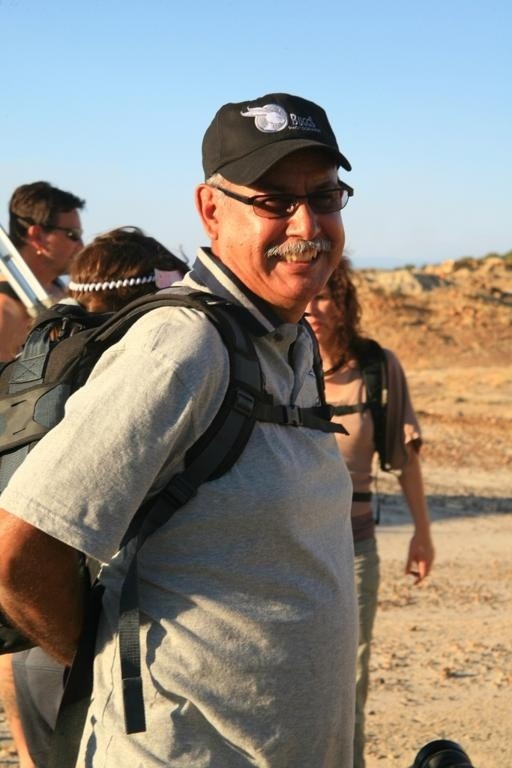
[23,223,87,242]
[206,174,356,222]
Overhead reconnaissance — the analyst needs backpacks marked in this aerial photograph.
[0,288,330,661]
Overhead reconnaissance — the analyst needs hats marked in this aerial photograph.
[199,90,352,188]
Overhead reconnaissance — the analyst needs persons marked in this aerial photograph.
[11,226,191,767]
[0,92,363,767]
[302,254,438,766]
[0,180,86,768]
